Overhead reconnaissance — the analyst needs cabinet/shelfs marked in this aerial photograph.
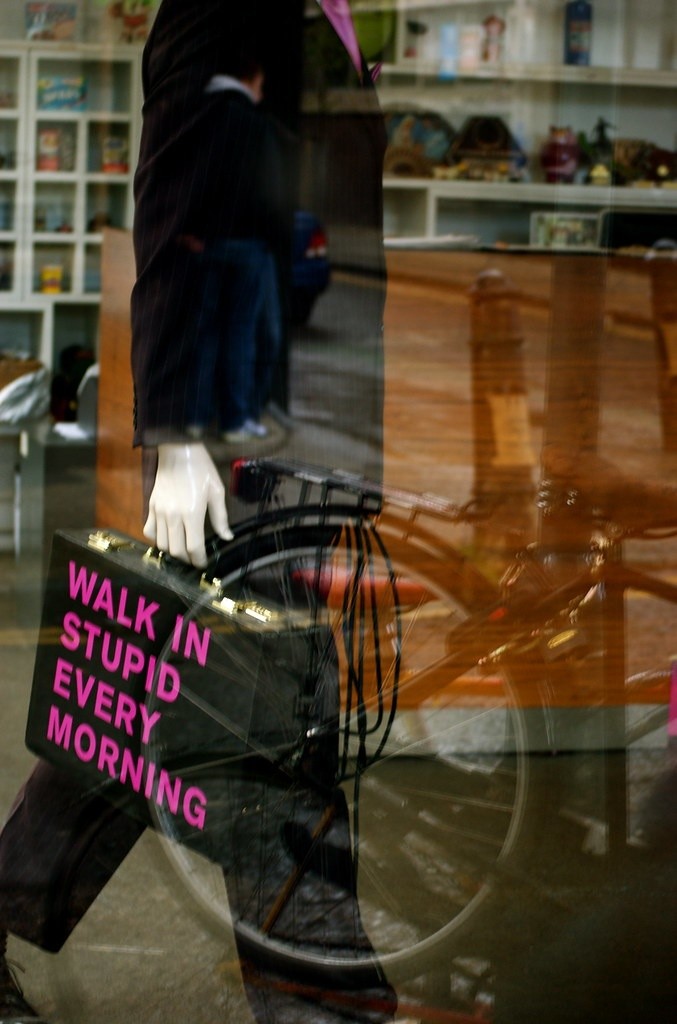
[0,0,677,302]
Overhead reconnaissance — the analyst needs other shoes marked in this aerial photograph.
[0,930,39,1024]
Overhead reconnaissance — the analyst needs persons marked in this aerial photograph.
[0,0,422,1024]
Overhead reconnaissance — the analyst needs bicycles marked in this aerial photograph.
[139,454,677,990]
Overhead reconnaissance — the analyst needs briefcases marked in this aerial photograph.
[24,527,341,882]
